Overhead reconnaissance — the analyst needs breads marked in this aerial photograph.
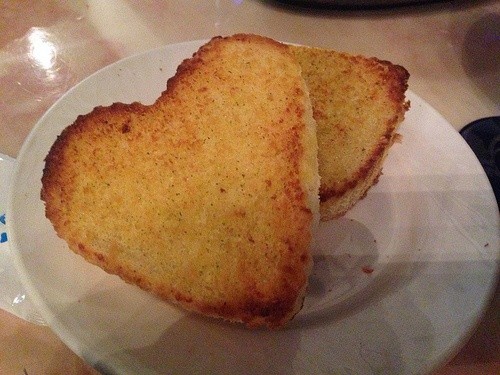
[38,33,411,332]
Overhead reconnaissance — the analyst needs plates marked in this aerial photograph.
[9,38,500,374]
[459,114,499,211]
